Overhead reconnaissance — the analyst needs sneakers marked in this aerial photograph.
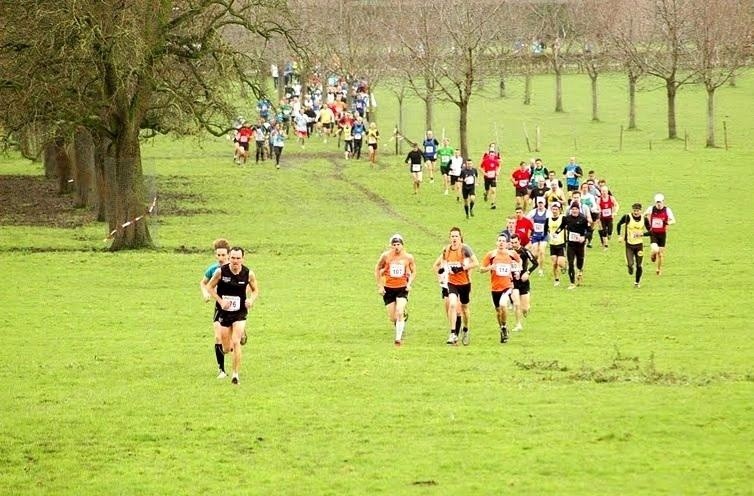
[512,325,523,332]
[217,371,228,379]
[628,265,634,275]
[240,332,246,345]
[523,308,529,318]
[403,311,408,322]
[500,327,508,343]
[634,282,639,288]
[656,270,661,276]
[446,328,469,346]
[231,376,239,385]
[539,267,582,289]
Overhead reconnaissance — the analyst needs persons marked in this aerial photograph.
[405,129,501,219]
[229,62,380,171]
[442,227,480,346]
[507,235,539,331]
[480,233,522,342]
[376,234,416,345]
[200,239,248,379]
[432,251,461,338]
[616,194,676,288]
[207,247,258,384]
[499,157,620,309]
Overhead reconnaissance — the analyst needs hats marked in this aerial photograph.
[655,193,664,203]
[538,197,546,204]
